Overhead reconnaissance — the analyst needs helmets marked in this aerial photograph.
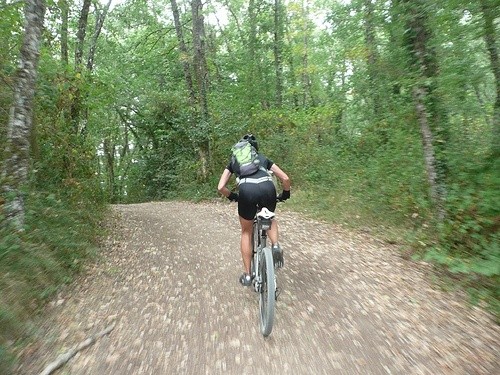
[239,134,259,151]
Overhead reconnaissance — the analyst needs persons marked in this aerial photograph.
[218,133,290,286]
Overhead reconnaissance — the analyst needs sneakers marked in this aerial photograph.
[272,247,284,270]
[239,273,252,286]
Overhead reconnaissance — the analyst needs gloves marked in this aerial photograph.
[228,191,239,202]
[278,190,291,202]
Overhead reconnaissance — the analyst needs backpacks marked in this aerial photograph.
[230,138,260,177]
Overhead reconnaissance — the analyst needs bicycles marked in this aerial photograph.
[252,198,286,337]
